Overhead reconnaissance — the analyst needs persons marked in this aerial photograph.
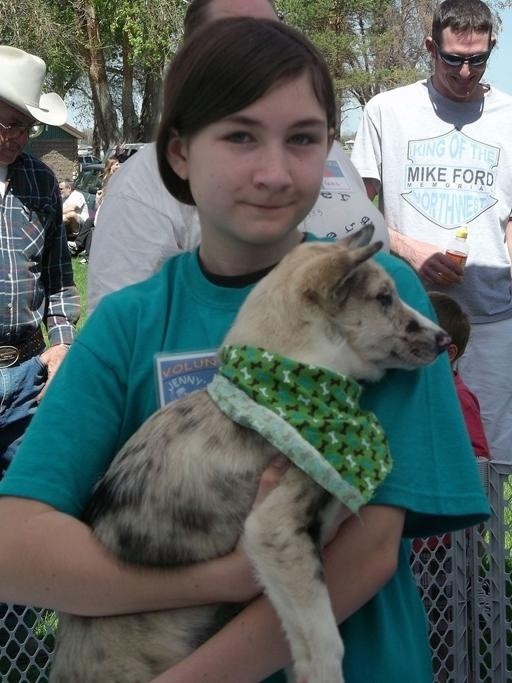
[425,291,492,460]
[67,158,121,255]
[350,0,512,552]
[59,180,89,237]
[85,0,388,324]
[0,15,490,682]
[0,41,80,478]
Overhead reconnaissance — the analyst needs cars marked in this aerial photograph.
[74,141,150,211]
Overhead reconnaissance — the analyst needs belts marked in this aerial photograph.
[0,324,45,369]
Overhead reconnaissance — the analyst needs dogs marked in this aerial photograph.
[47,223,453,683]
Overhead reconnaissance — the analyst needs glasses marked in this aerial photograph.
[433,40,491,68]
[0,123,48,140]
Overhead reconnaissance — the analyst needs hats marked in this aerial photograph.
[0,45,67,127]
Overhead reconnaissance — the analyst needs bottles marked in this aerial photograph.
[443,229,469,278]
